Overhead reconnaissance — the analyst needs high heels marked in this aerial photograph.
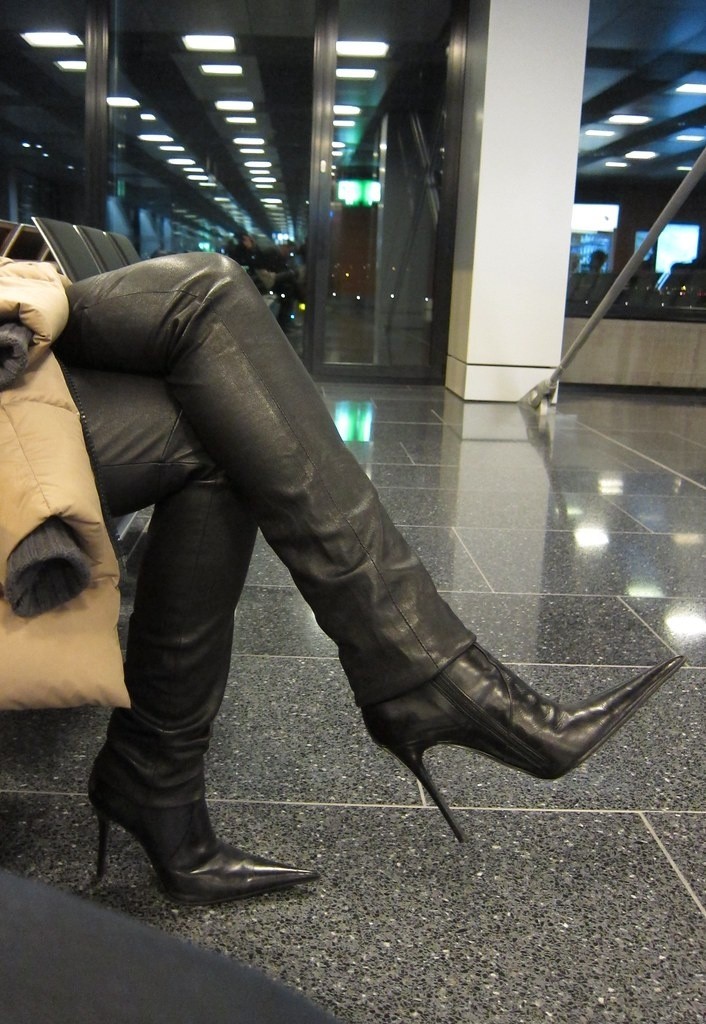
[361,642,688,844]
[89,773,321,904]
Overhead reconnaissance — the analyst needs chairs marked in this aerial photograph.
[2,216,279,320]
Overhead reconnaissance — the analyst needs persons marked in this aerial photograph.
[568,250,607,299]
[227,232,308,334]
[56,255,687,902]
